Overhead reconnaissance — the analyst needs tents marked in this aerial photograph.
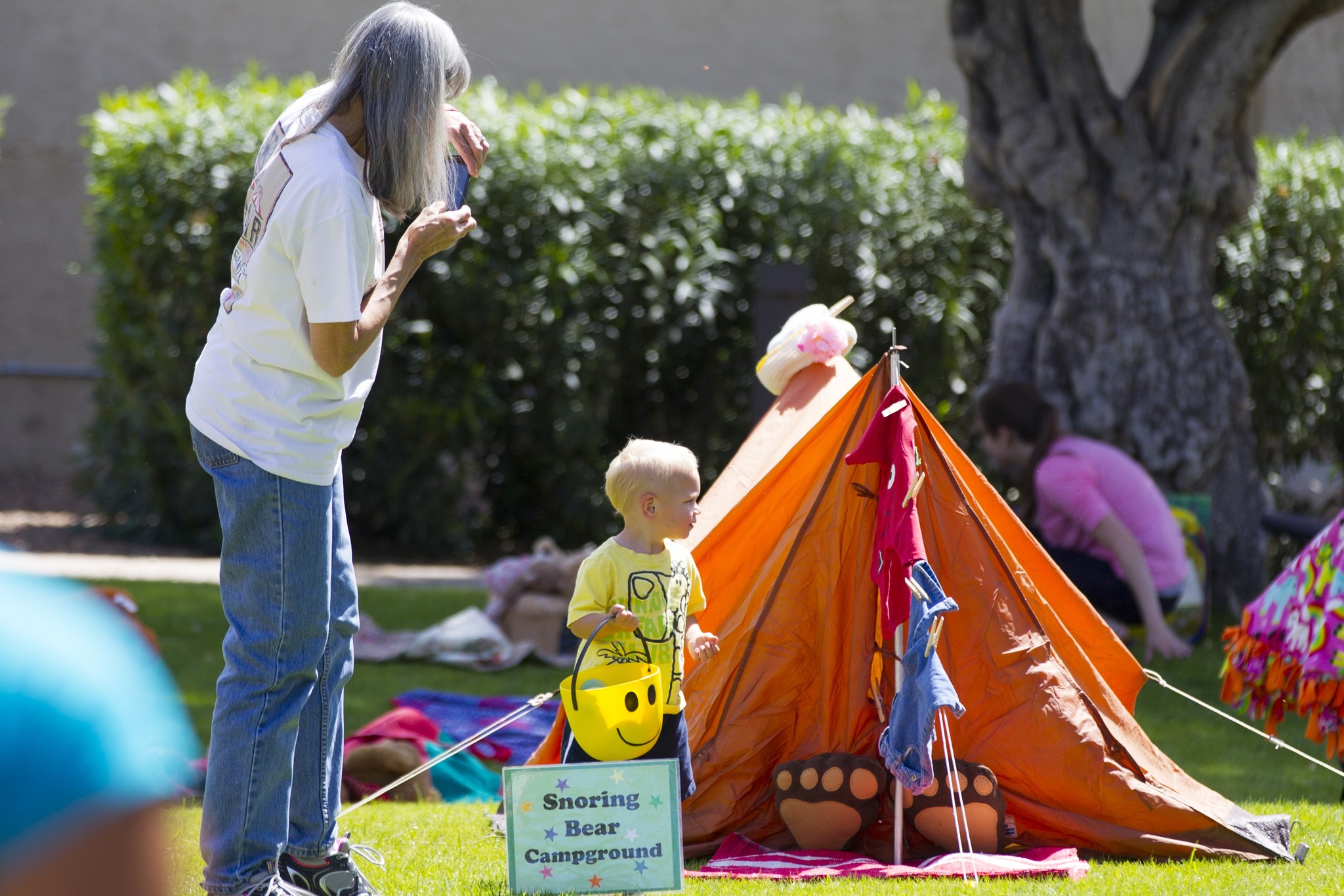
[490,335,1297,873]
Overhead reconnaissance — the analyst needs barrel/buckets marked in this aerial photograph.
[560,614,663,760]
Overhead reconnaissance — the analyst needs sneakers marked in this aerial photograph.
[206,879,292,896]
[276,832,376,896]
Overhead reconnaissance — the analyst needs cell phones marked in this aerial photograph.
[428,155,471,214]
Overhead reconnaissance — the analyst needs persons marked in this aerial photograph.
[556,438,726,802]
[0,573,202,892]
[183,0,491,896]
[971,377,1193,667]
